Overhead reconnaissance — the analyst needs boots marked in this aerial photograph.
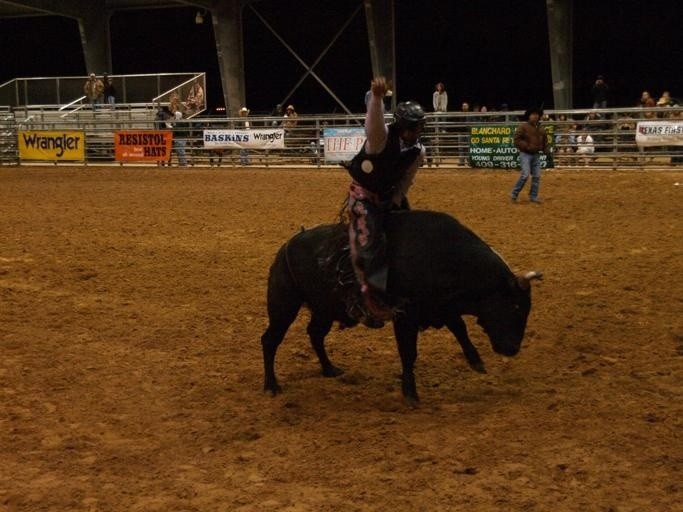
[361,285,392,319]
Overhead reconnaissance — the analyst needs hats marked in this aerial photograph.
[523,106,543,121]
[239,107,250,116]
[657,97,669,105]
[287,105,294,110]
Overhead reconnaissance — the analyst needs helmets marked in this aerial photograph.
[393,101,426,124]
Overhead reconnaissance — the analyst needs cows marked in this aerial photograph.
[259,209,544,402]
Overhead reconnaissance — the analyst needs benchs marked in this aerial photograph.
[168,128,683,168]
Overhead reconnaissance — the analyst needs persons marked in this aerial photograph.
[475,105,489,122]
[168,106,191,167]
[454,102,473,166]
[493,103,520,124]
[591,75,609,108]
[233,107,252,165]
[417,127,443,170]
[205,112,225,169]
[509,106,551,205]
[82,73,104,107]
[168,79,206,122]
[280,104,298,128]
[432,83,449,134]
[539,88,682,169]
[101,72,118,112]
[149,106,174,168]
[339,76,429,318]
[271,104,283,128]
